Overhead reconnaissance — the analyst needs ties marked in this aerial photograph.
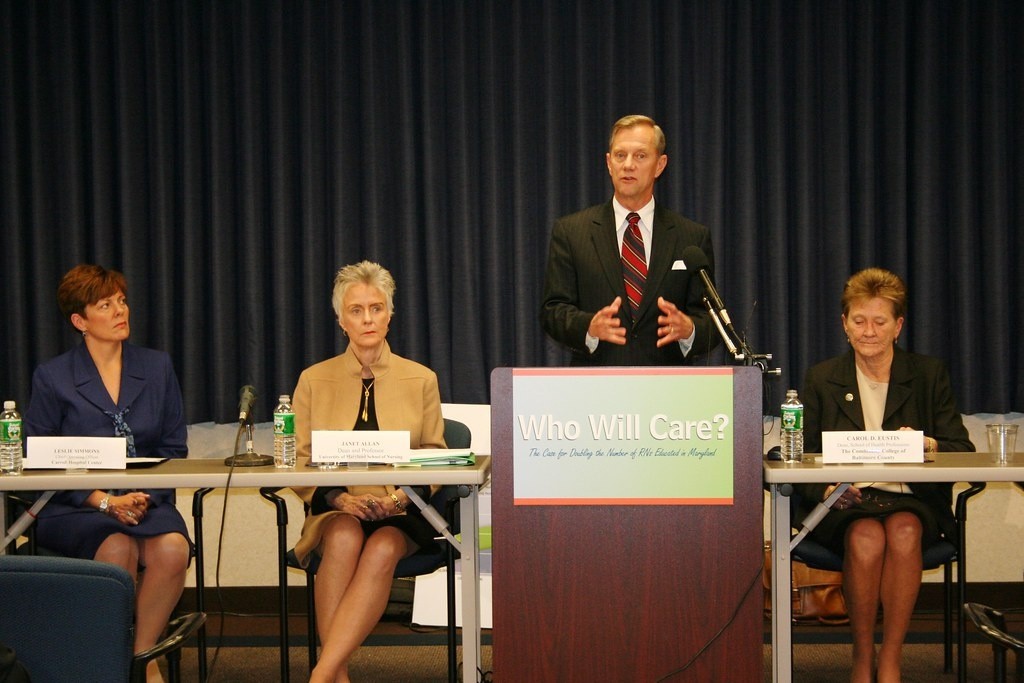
[621,213,648,323]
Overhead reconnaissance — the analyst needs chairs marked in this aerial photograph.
[1,464,209,683]
[260,420,471,683]
[765,446,988,683]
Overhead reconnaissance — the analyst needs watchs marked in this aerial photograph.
[99,494,111,512]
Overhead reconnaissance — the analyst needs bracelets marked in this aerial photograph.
[388,493,402,510]
[927,437,934,452]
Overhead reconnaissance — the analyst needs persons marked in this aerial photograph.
[21,264,194,683]
[799,268,976,683]
[281,260,450,683]
[538,115,725,367]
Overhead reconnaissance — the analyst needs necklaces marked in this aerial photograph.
[362,380,374,422]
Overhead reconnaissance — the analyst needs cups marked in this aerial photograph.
[348,462,368,469]
[986,424,1018,464]
[317,461,339,469]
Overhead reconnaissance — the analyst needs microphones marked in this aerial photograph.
[682,245,736,331]
[238,385,258,425]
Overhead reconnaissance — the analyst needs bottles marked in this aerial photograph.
[779,390,804,462]
[0,401,23,476]
[273,395,297,468]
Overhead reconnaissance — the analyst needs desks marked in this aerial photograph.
[0,458,490,683]
[764,453,1024,683]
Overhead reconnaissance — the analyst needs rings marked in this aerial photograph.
[840,504,843,510]
[361,506,368,512]
[843,499,848,504]
[127,511,133,516]
[671,327,674,334]
[367,499,375,506]
